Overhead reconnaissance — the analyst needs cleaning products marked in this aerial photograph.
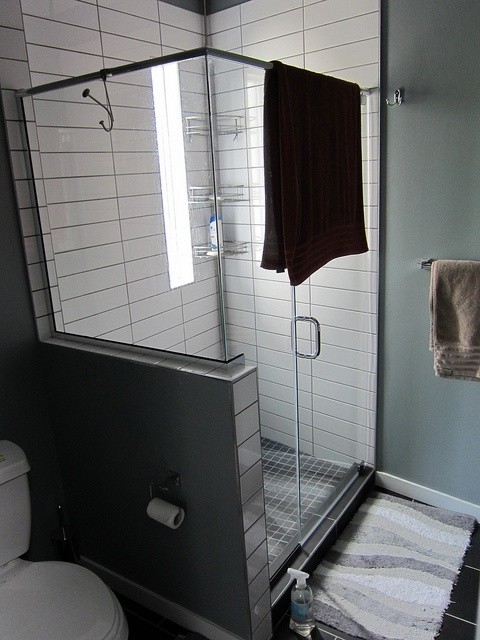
[286,566,316,638]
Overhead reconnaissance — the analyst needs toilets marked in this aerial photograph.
[0,439,129,640]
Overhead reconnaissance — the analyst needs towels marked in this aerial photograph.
[259,59,369,286]
[427,258,480,383]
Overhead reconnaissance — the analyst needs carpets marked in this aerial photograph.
[308,487,476,639]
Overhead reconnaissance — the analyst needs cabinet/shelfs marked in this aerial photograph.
[186,114,249,259]
